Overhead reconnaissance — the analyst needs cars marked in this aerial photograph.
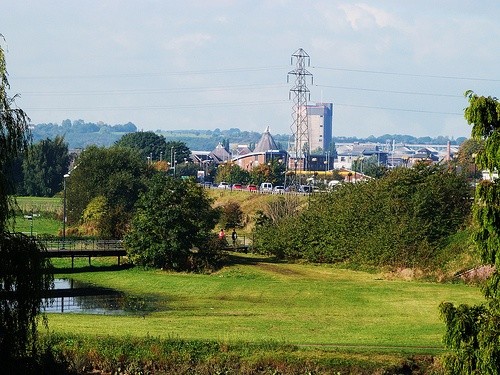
[203,181,313,197]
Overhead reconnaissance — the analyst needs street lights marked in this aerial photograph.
[62,173,70,249]
